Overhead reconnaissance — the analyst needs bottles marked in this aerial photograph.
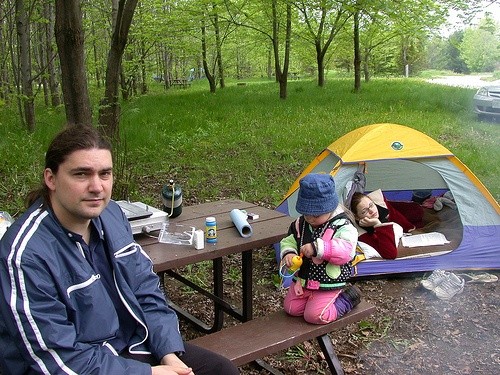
[205,216,217,243]
[161,178,184,218]
[287,256,302,275]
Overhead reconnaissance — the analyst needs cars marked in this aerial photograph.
[473,79,500,118]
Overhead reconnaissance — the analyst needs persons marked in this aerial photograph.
[279,173,361,324]
[350,190,426,259]
[0,124,240,375]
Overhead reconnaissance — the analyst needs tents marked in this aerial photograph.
[273,123,500,277]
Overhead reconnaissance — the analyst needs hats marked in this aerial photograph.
[295,173,339,216]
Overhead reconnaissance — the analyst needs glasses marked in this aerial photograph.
[357,202,375,216]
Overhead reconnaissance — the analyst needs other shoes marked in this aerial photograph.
[344,285,362,308]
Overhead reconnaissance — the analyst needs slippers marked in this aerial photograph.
[457,272,499,283]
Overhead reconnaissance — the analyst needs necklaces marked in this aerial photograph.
[298,221,329,255]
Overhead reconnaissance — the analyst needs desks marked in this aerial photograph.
[135,198,296,332]
[172,79,187,87]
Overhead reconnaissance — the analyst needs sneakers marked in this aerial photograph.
[420,269,451,291]
[431,273,465,301]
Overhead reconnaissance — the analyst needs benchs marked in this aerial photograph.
[170,82,193,89]
[188,298,376,375]
